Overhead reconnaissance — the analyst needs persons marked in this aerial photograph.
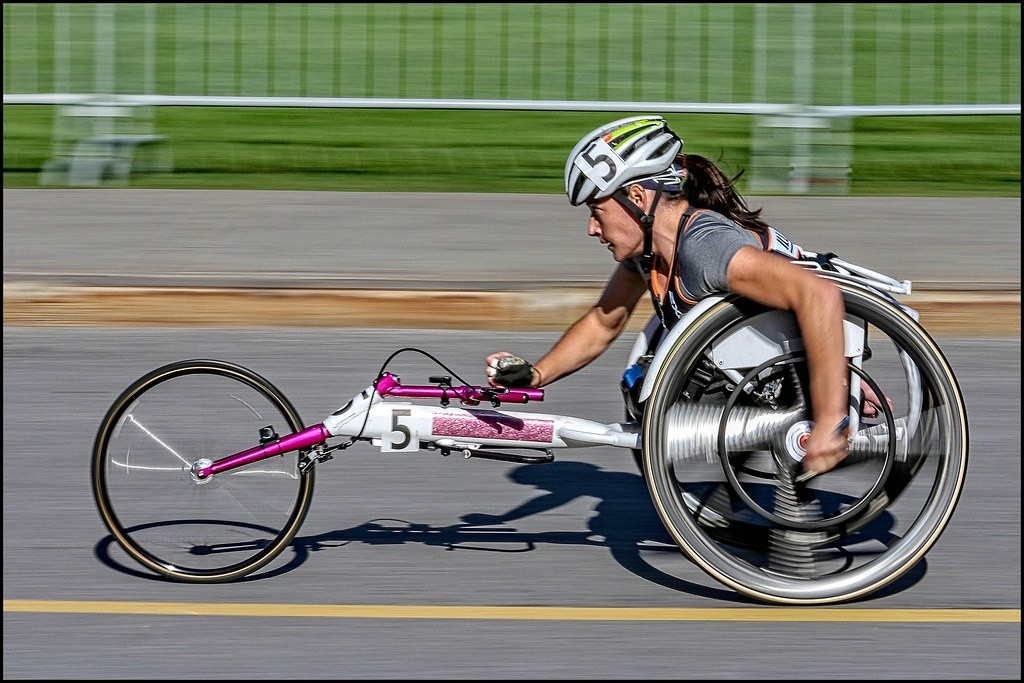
[486,116,896,472]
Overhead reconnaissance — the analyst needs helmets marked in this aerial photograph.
[563,115,685,208]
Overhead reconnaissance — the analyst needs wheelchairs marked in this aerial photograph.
[88,248,971,608]
